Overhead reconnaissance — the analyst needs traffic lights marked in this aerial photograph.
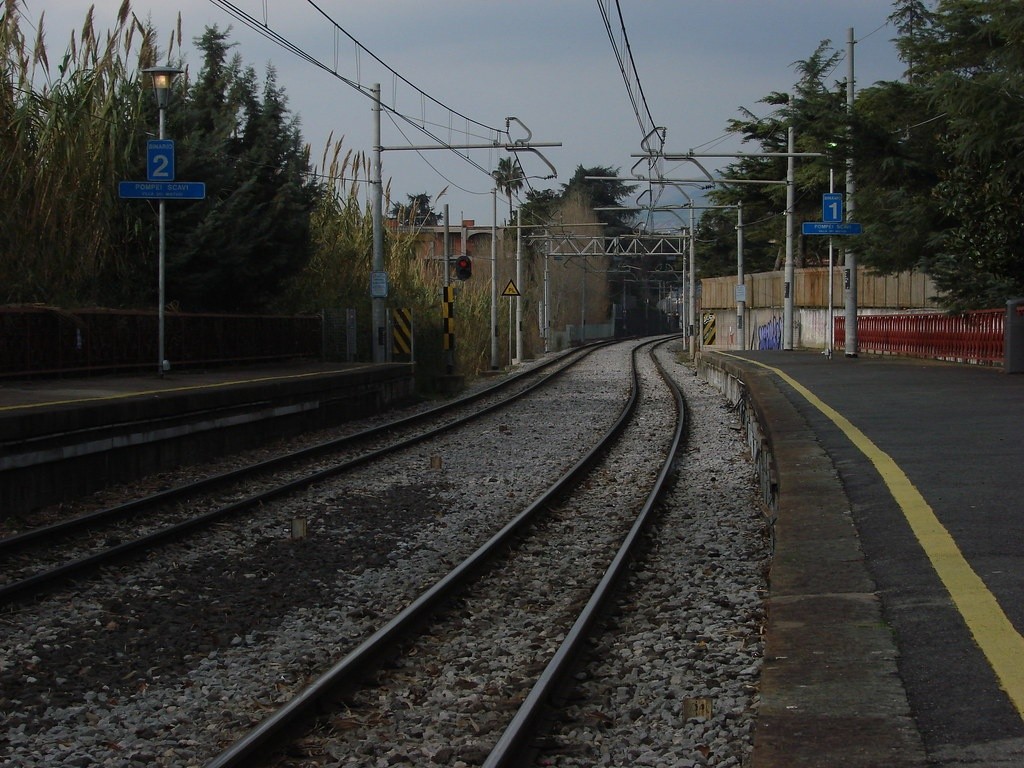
[456,256,471,281]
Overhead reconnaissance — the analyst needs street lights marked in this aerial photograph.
[140,66,185,379]
[818,133,851,360]
